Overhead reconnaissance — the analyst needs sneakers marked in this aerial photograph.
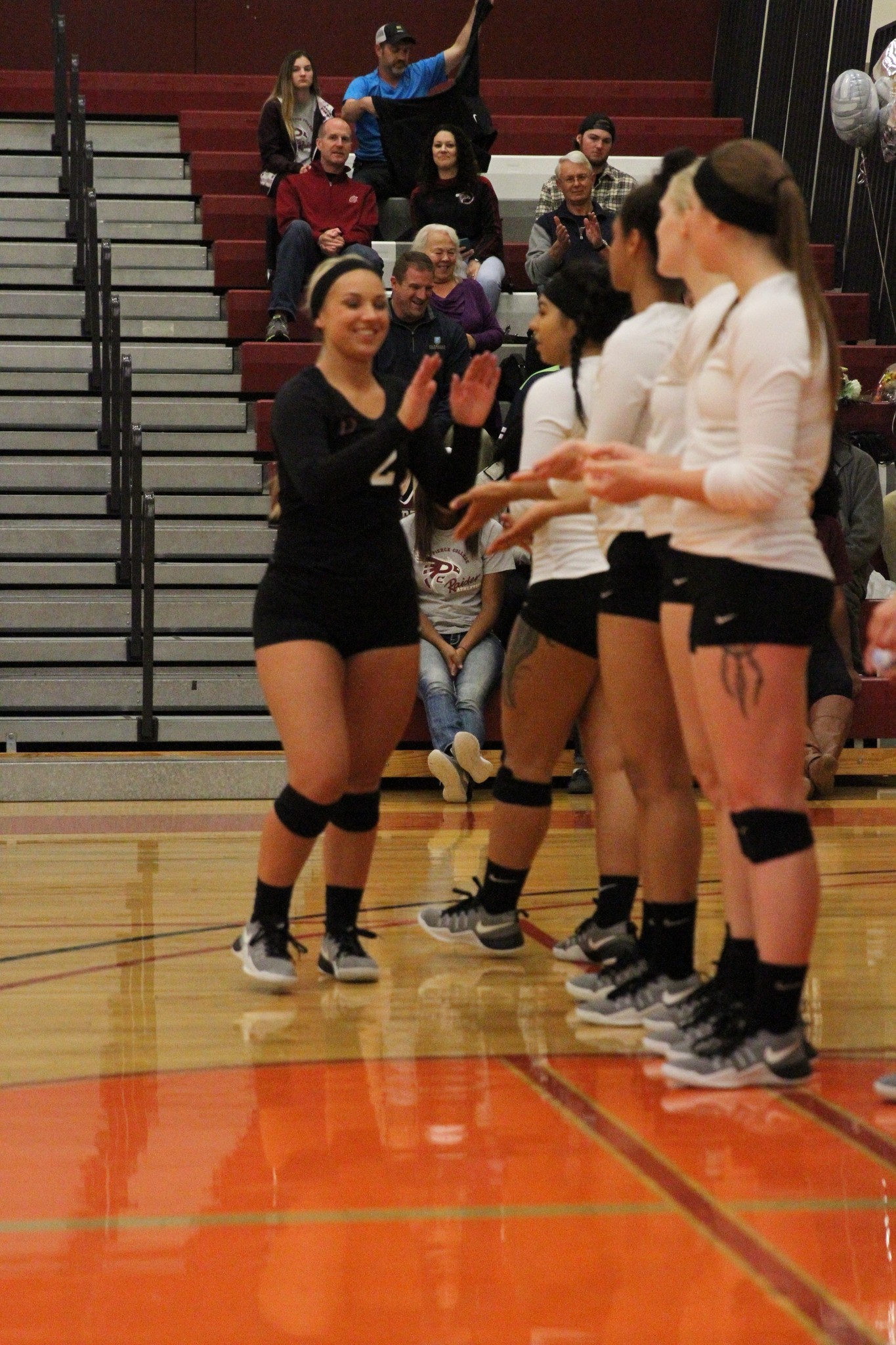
[566,945,654,1002]
[417,876,529,952]
[660,1027,812,1088]
[642,977,725,1027]
[316,925,379,982]
[642,992,742,1052]
[664,1014,817,1059]
[552,898,638,964]
[231,912,308,983]
[575,969,703,1025]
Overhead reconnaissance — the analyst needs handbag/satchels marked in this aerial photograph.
[495,353,529,401]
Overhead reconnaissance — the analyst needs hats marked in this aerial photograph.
[579,114,615,139]
[375,23,416,45]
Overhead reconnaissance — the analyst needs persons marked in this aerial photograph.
[266,117,380,340]
[580,139,837,1086]
[524,152,616,292]
[410,124,507,315]
[228,1,895,1099]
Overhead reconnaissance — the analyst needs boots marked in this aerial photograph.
[803,725,837,796]
[803,695,855,800]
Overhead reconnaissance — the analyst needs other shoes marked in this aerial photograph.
[265,314,290,342]
[568,769,591,793]
[450,731,493,783]
[428,749,469,803]
[872,1074,896,1097]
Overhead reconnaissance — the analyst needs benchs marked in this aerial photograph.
[177,108,896,748]
